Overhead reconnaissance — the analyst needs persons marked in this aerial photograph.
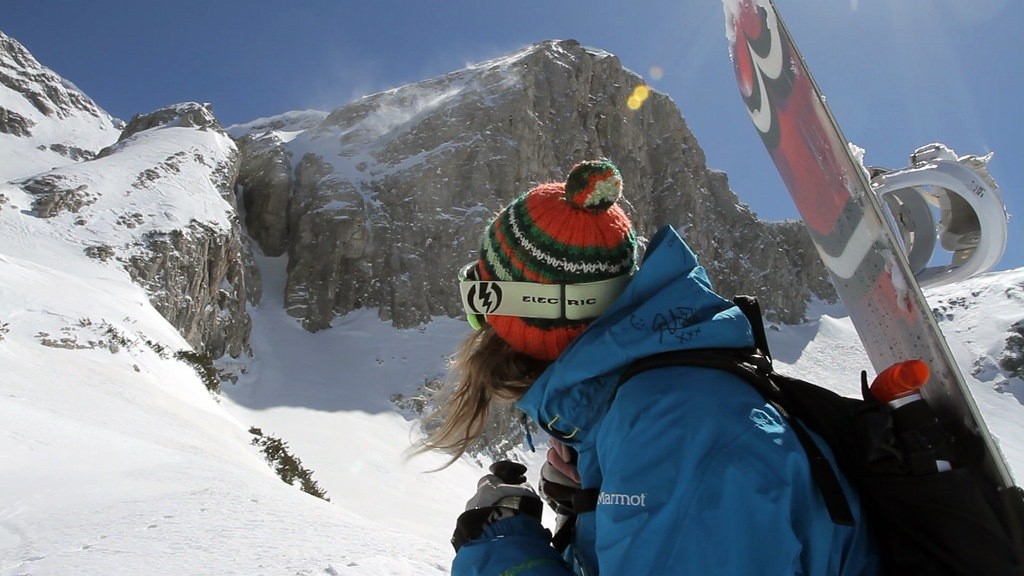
[450,158,882,576]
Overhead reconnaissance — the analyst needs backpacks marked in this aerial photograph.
[607,291,1024,575]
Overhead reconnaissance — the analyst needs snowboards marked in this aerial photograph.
[723,0,1014,487]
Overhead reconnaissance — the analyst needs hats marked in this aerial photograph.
[470,160,645,363]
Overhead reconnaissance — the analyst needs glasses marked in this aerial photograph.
[458,259,492,331]
